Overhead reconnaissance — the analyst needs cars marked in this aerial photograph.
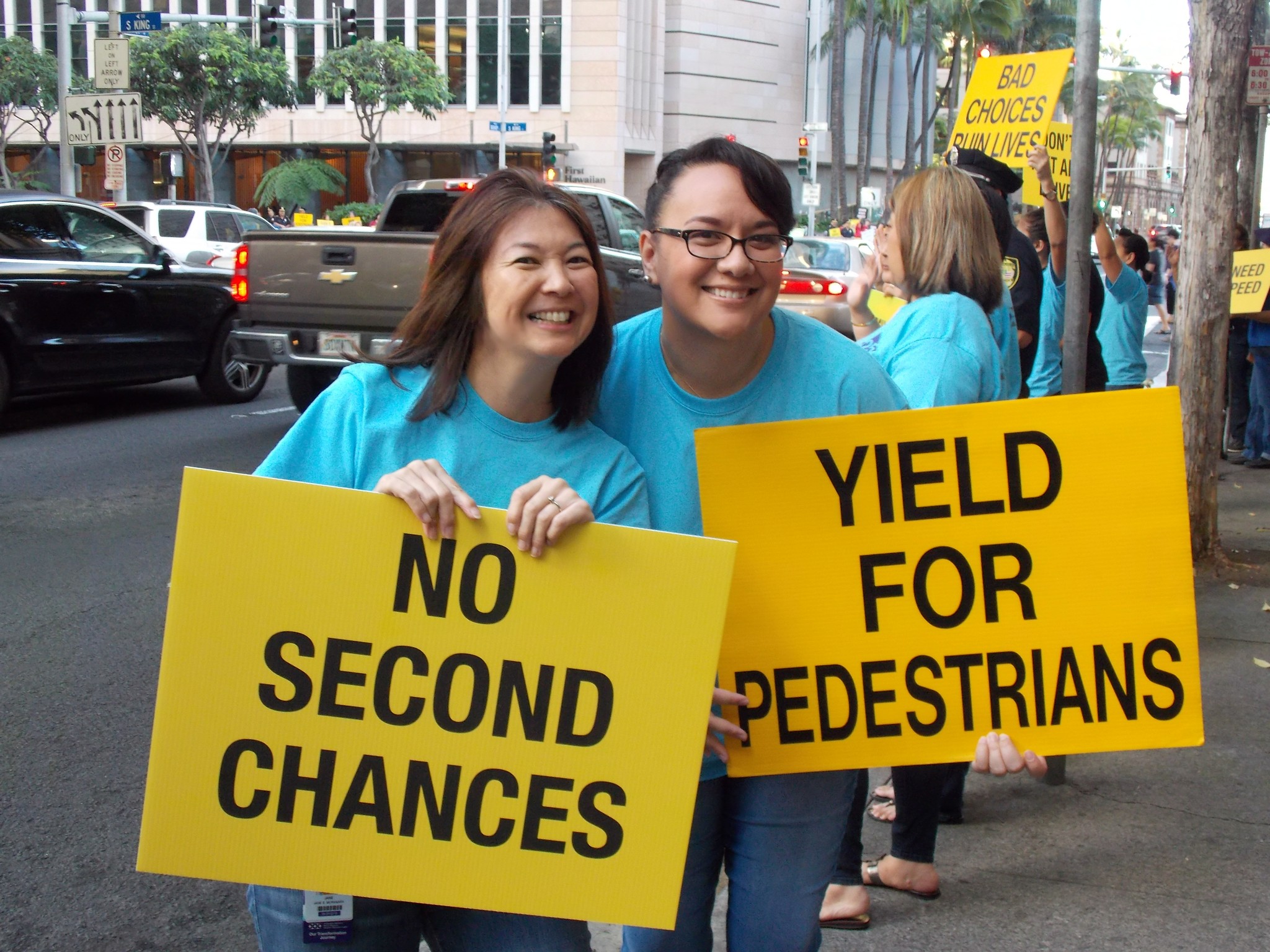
[1090,224,1122,258]
[1147,224,1182,247]
[0,190,273,405]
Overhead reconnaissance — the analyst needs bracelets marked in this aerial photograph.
[850,314,877,326]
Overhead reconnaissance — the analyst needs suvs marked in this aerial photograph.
[69,199,282,276]
[775,235,877,338]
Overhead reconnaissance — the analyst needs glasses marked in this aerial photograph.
[651,227,794,264]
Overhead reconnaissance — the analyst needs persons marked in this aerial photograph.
[818,163,1270,931]
[1090,194,1150,394]
[589,138,1047,952]
[253,206,315,228]
[247,167,653,952]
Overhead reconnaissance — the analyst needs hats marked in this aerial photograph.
[945,146,1025,195]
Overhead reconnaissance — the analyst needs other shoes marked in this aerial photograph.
[1228,452,1270,467]
[1156,328,1172,334]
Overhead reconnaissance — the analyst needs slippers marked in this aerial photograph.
[869,789,899,800]
[818,914,870,930]
[863,853,942,899]
[867,803,899,822]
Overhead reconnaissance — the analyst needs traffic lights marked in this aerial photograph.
[1098,200,1106,209]
[542,133,557,164]
[726,133,735,142]
[796,136,809,176]
[336,6,357,49]
[77,146,96,167]
[160,150,187,184]
[1170,66,1181,94]
[978,47,992,59]
[252,4,277,49]
[1166,166,1171,178]
[1169,207,1175,214]
[548,168,561,181]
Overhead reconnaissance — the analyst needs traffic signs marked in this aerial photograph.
[65,92,143,147]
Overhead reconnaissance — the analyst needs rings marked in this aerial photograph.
[548,494,562,512]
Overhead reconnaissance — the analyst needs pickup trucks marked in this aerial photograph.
[230,176,664,417]
